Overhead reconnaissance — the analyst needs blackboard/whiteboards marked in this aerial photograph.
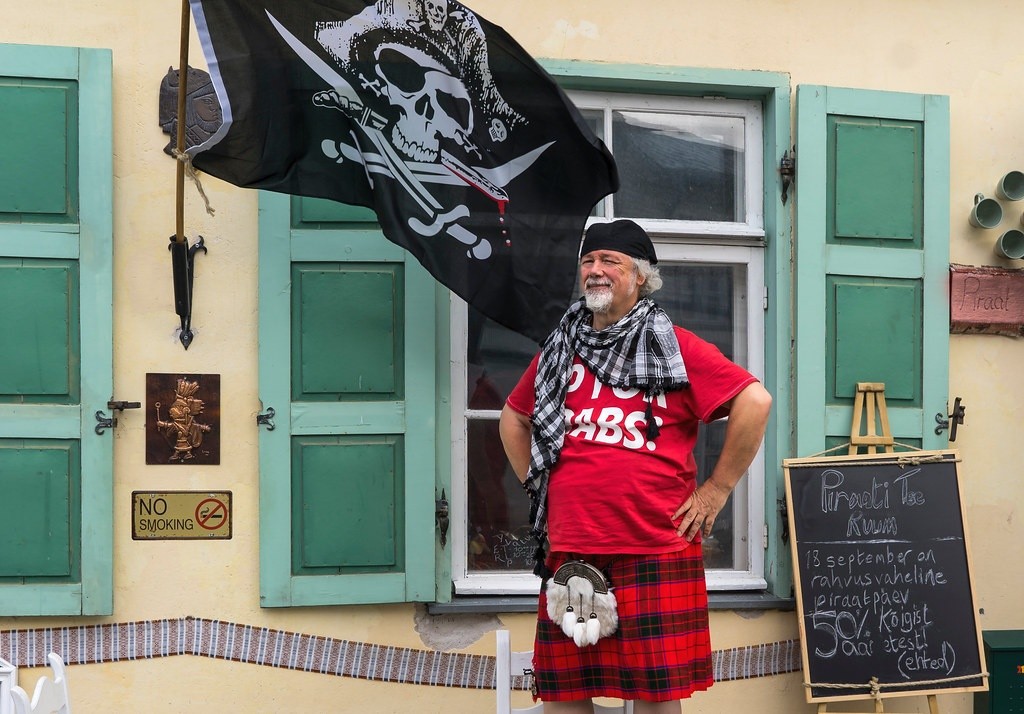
[781,448,990,704]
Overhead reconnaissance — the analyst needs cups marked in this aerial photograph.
[968,193,1003,229]
[995,170,1024,201]
[993,229,1024,259]
[1020,212,1024,225]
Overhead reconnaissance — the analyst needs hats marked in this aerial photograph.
[580,219,659,265]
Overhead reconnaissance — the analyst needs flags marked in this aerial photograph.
[176,0,620,344]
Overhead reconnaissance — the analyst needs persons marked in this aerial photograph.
[499,218,773,714]
